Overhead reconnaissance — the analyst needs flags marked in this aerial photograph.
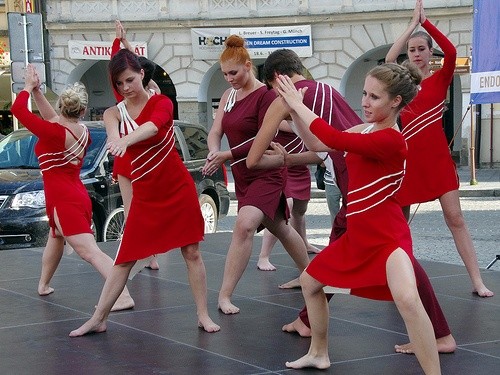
[469,0,500,104]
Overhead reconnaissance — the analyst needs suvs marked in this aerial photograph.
[0,120,229,252]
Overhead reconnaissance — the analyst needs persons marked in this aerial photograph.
[384,0,494,298]
[108,18,161,270]
[67,50,222,337]
[256,64,322,272]
[245,49,456,354]
[275,61,441,375]
[10,63,134,312]
[201,34,312,315]
